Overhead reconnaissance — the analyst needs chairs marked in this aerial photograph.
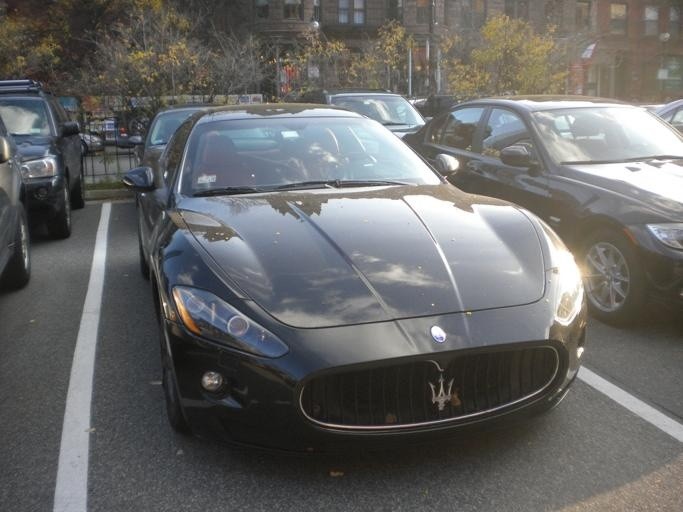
[571,115,607,160]
[194,129,260,188]
